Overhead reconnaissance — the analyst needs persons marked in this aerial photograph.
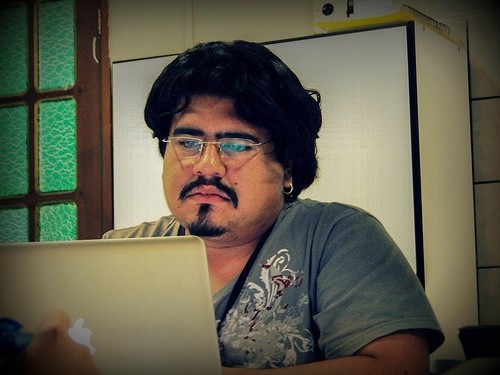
[23,306,100,375]
[100,39,446,375]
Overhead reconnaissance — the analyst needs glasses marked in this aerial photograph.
[161,136,274,162]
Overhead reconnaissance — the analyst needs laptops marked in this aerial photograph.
[0,235,224,375]
